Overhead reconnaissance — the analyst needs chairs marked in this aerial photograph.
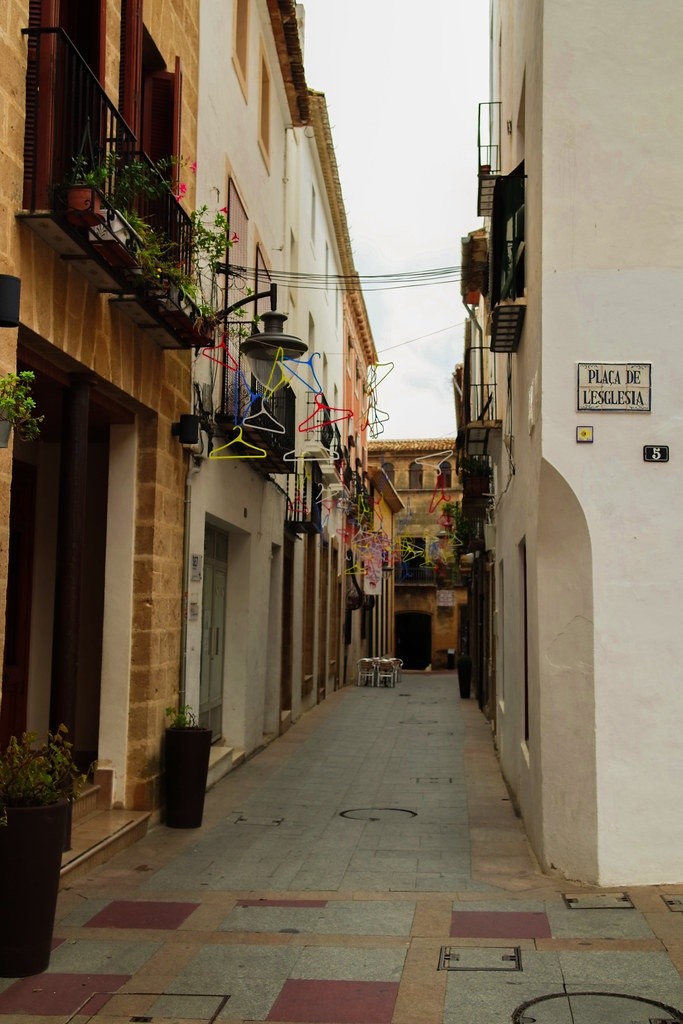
[357,657,403,687]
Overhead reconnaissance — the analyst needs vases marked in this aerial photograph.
[89,210,129,246]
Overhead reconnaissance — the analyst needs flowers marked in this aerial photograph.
[89,156,196,210]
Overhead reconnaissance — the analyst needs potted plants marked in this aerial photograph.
[65,150,125,226]
[129,204,238,344]
[0,371,44,448]
[0,723,99,977]
[458,457,493,495]
[166,705,213,828]
[457,655,472,698]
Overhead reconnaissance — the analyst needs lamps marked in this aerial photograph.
[0,273,21,328]
[207,283,308,391]
[436,522,453,539]
[171,413,199,444]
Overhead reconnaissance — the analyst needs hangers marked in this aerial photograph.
[203,333,464,581]
